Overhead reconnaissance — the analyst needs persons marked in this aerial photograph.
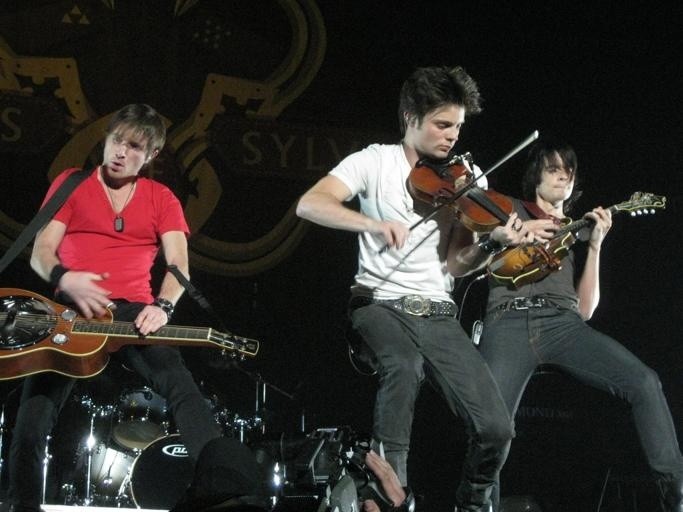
[295,66,532,511]
[8,102,221,512]
[475,141,682,511]
[360,448,406,511]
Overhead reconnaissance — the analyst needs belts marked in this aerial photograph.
[368,294,455,317]
[496,297,555,310]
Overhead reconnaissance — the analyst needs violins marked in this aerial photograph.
[406,151,563,273]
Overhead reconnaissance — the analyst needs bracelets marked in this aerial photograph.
[48,264,68,288]
[150,297,174,321]
[478,231,506,258]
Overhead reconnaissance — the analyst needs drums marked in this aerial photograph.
[82,385,173,501]
[223,413,262,450]
[115,434,197,508]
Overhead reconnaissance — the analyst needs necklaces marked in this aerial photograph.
[105,181,135,231]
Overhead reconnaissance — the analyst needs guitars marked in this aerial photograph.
[486,191,667,292]
[0,288,259,382]
